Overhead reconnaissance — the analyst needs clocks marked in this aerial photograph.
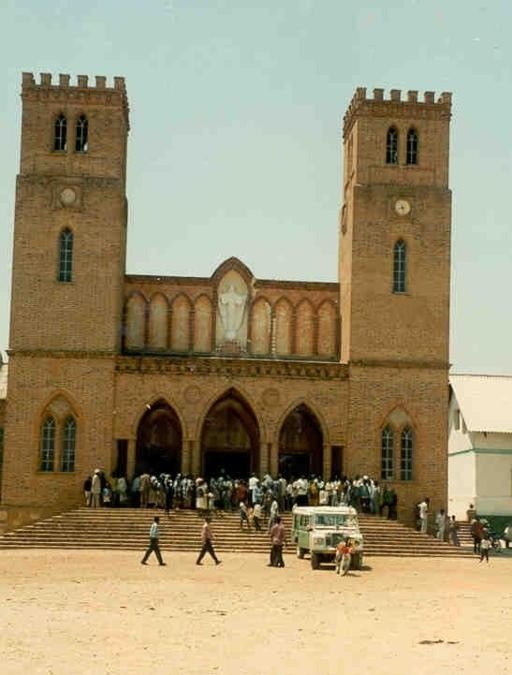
[391,198,413,218]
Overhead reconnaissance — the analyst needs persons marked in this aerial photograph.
[83,466,493,567]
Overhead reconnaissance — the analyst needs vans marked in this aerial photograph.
[290,506,363,570]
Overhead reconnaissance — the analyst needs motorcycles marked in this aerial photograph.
[333,533,357,579]
